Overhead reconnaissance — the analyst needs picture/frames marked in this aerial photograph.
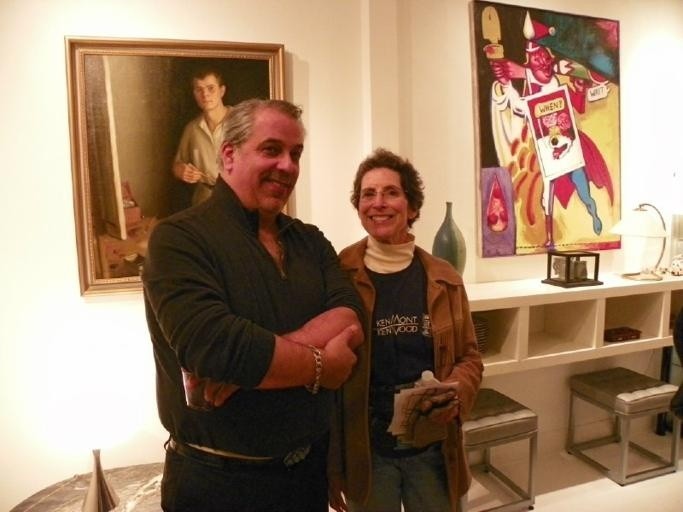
[65,35,298,299]
[469,0,622,257]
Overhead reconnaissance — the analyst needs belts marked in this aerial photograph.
[167,435,328,470]
[201,182,216,191]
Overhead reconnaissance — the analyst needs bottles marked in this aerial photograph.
[414,370,440,388]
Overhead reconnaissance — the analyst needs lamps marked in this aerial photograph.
[613,202,668,280]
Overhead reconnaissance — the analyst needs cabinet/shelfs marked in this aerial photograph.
[461,268,682,380]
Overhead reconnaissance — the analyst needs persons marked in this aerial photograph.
[326,145,484,512]
[139,96,367,511]
[168,66,235,208]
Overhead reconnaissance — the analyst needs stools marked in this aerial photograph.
[458,388,537,511]
[568,368,680,486]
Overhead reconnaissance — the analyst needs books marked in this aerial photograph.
[386,379,460,452]
[604,326,643,343]
[471,314,489,356]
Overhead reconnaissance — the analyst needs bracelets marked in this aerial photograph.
[303,342,324,395]
